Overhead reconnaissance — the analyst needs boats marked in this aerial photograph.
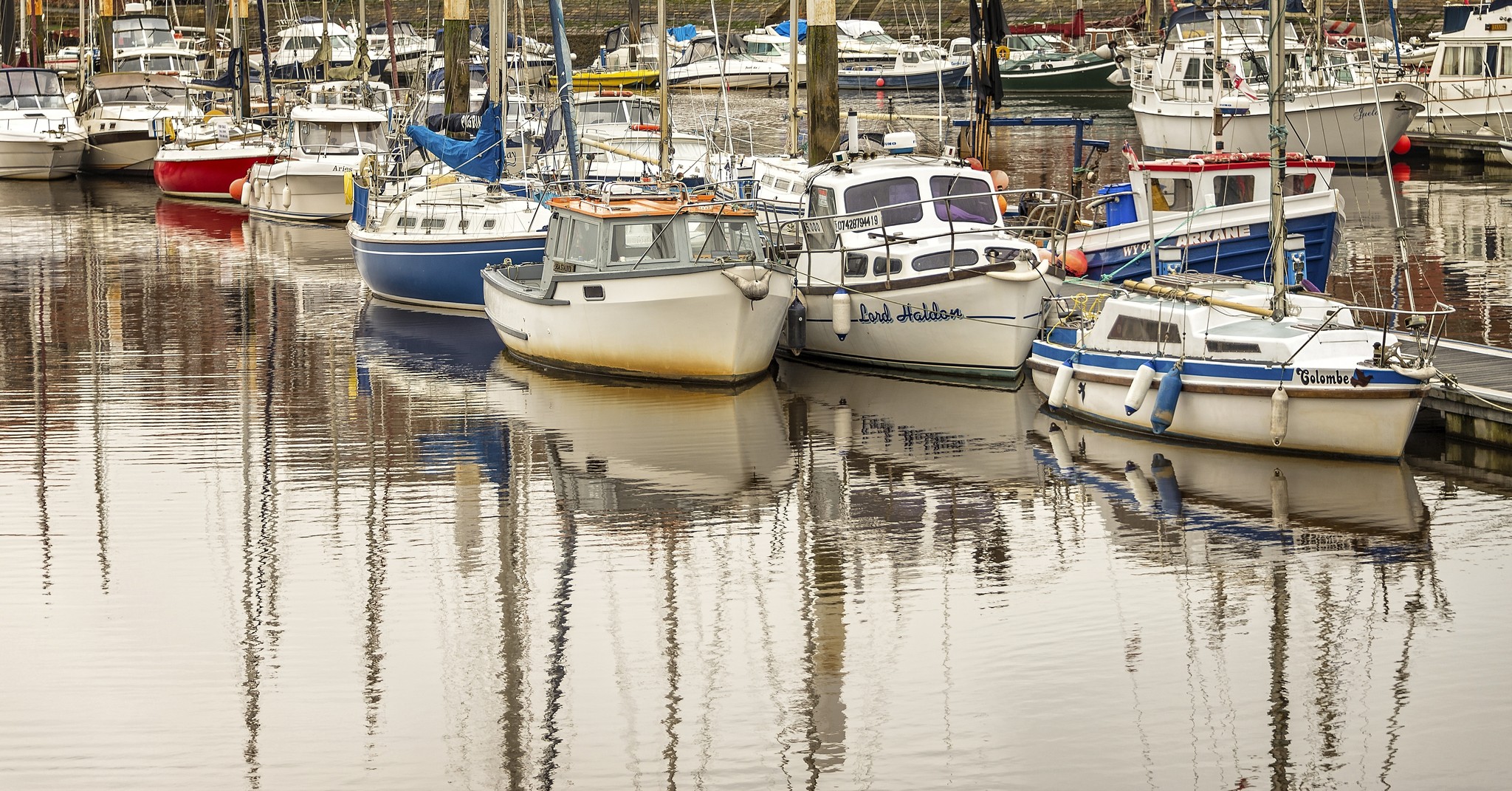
[1024,1,1456,465]
[0,0,1512,390]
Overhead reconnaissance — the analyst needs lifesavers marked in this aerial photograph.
[174,33,182,39]
[343,172,353,204]
[631,124,661,132]
[595,91,633,97]
[203,109,225,123]
[360,154,382,187]
[995,46,1011,59]
[156,71,180,76]
[164,117,176,141]
